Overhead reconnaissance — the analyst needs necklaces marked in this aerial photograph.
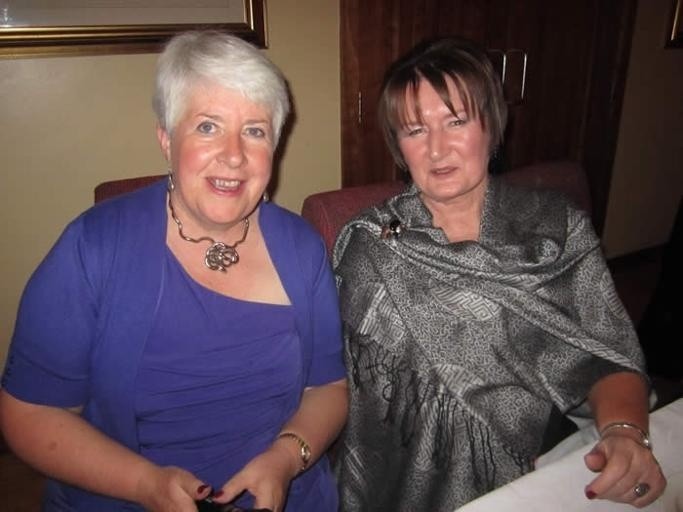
[167,189,250,272]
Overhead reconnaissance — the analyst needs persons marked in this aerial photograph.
[0,31,348,512]
[332,40,667,512]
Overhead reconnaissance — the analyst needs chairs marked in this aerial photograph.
[300,162,595,275]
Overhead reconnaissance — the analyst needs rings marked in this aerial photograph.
[633,483,649,497]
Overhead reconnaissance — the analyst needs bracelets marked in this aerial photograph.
[598,421,653,446]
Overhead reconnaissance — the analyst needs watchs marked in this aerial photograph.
[271,432,312,477]
